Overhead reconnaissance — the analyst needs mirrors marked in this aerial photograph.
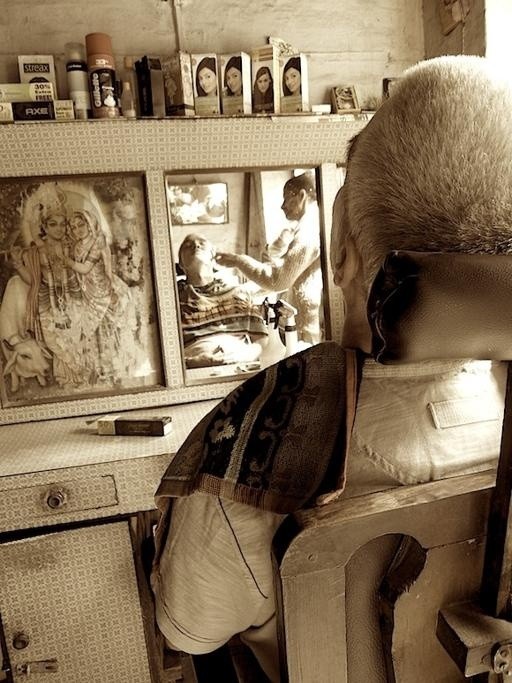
[166,164,334,386]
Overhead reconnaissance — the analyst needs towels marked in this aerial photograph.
[153,340,364,567]
[176,278,268,347]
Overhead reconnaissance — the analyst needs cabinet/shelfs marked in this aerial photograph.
[0,115,404,427]
[1,399,325,683]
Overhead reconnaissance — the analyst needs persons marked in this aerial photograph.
[214,173,324,347]
[2,182,153,400]
[151,54,511,683]
[282,57,301,97]
[165,70,178,105]
[222,55,243,95]
[196,57,217,97]
[177,233,270,369]
[253,67,274,112]
[170,186,224,224]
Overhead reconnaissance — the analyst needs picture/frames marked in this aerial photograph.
[168,182,229,225]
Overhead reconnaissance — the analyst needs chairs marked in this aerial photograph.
[161,250,507,683]
